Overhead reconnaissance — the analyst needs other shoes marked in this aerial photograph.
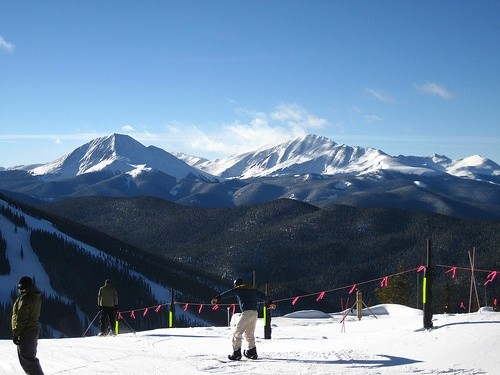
[109,331,116,336]
[98,332,104,337]
[244,347,257,359]
[228,348,241,361]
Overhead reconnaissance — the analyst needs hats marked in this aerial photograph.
[234,279,242,285]
[105,279,111,283]
[19,276,33,287]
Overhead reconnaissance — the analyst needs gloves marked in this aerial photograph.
[12,334,21,346]
[114,305,118,310]
[98,306,101,311]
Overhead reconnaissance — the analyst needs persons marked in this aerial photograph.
[12,276,44,375]
[98,279,118,337]
[211,278,276,360]
